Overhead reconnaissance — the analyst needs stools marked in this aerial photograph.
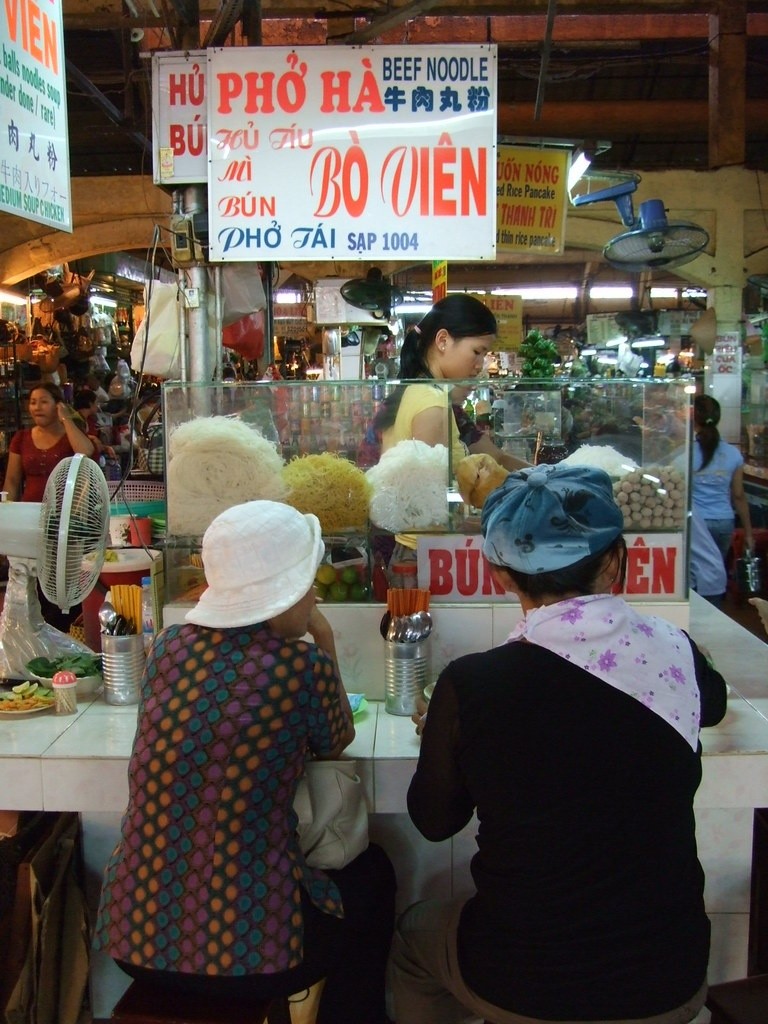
[88,962,295,1024]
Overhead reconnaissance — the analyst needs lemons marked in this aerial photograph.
[3,679,52,700]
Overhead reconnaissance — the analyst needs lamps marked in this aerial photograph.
[567,148,598,193]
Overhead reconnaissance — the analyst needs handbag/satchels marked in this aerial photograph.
[2,260,94,374]
[212,262,267,327]
[221,309,265,362]
[130,227,188,379]
[291,756,372,871]
[137,401,164,473]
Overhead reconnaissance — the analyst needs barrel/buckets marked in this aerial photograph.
[80,549,160,653]
[737,548,762,593]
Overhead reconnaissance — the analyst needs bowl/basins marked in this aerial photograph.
[28,670,102,696]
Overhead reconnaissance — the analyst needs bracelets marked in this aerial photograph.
[61,416,72,420]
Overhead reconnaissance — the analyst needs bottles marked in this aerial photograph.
[141,577,154,657]
[653,362,665,377]
[464,400,474,423]
[117,307,130,331]
[389,562,417,589]
[606,365,616,379]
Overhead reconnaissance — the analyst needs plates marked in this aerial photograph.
[345,693,369,716]
[148,512,166,533]
[0,692,55,713]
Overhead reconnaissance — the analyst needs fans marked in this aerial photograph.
[616,302,652,334]
[341,267,404,319]
[34,273,81,313]
[370,358,395,379]
[0,452,110,690]
[572,178,709,272]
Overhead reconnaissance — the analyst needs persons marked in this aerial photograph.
[91,500,398,1024]
[384,464,729,1024]
[358,294,537,603]
[0,385,102,630]
[594,394,756,608]
[70,389,118,480]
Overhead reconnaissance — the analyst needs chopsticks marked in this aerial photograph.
[387,588,430,617]
[110,584,143,635]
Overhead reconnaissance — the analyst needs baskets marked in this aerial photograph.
[103,480,166,506]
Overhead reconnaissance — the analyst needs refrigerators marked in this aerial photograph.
[341,330,366,380]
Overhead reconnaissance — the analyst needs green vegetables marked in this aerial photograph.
[24,651,103,678]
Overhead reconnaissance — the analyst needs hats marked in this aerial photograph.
[479,463,624,576]
[184,500,326,629]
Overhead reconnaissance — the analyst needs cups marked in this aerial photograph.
[101,632,145,706]
[130,518,151,547]
[384,640,429,716]
[422,682,436,706]
[110,515,137,547]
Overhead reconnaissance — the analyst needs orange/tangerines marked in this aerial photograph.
[311,565,369,602]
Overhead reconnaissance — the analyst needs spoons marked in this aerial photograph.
[380,611,433,642]
[98,602,135,635]
